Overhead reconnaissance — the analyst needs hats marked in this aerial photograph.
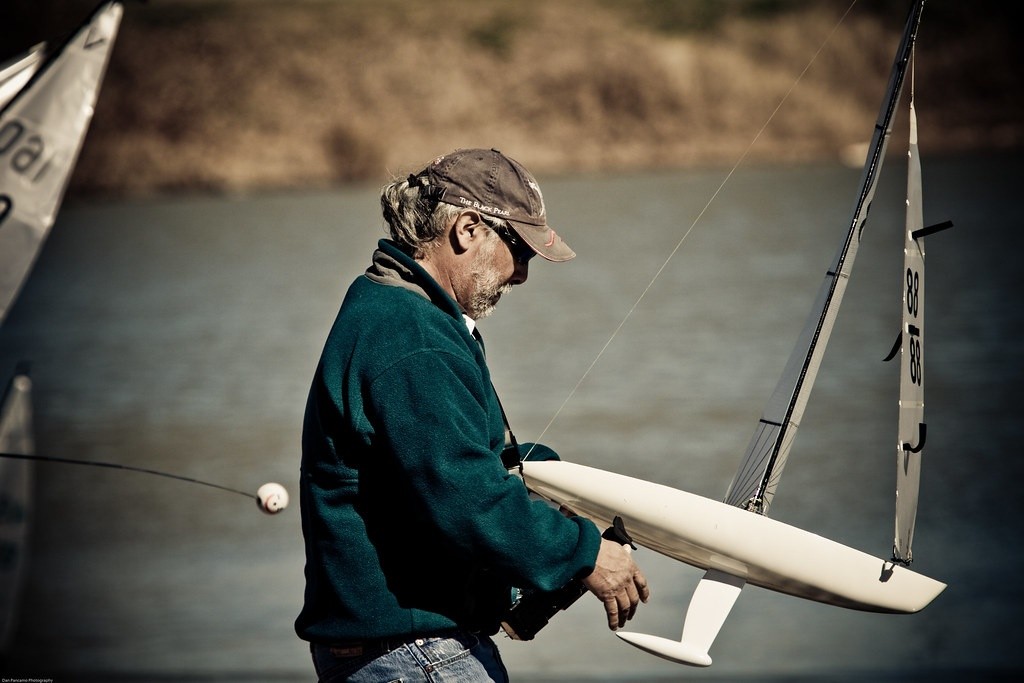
[424,148,576,262]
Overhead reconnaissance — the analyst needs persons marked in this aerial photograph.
[294,148,649,683]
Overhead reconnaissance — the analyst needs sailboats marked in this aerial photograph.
[495,1,951,669]
[0,0,126,637]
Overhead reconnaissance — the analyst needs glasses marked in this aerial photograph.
[479,215,537,266]
